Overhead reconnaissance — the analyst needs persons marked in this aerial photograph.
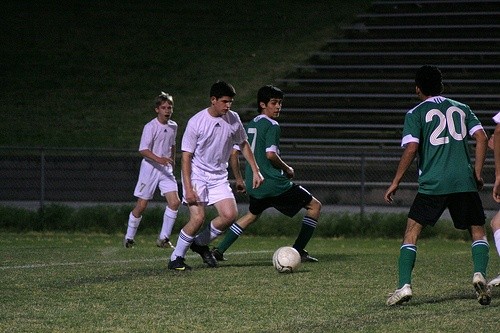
[123,91,181,249]
[167,81,265,271]
[486,111,500,287]
[211,85,322,262]
[384,65,492,307]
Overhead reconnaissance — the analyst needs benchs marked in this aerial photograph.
[238,0,500,210]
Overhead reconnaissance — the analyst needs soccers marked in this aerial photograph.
[273,246,301,273]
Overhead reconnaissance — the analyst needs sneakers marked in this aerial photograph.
[212,248,228,261]
[301,249,318,263]
[156,237,175,249]
[124,239,136,249]
[385,284,413,307]
[190,239,217,267]
[488,274,500,287]
[471,271,492,306]
[168,256,191,272]
